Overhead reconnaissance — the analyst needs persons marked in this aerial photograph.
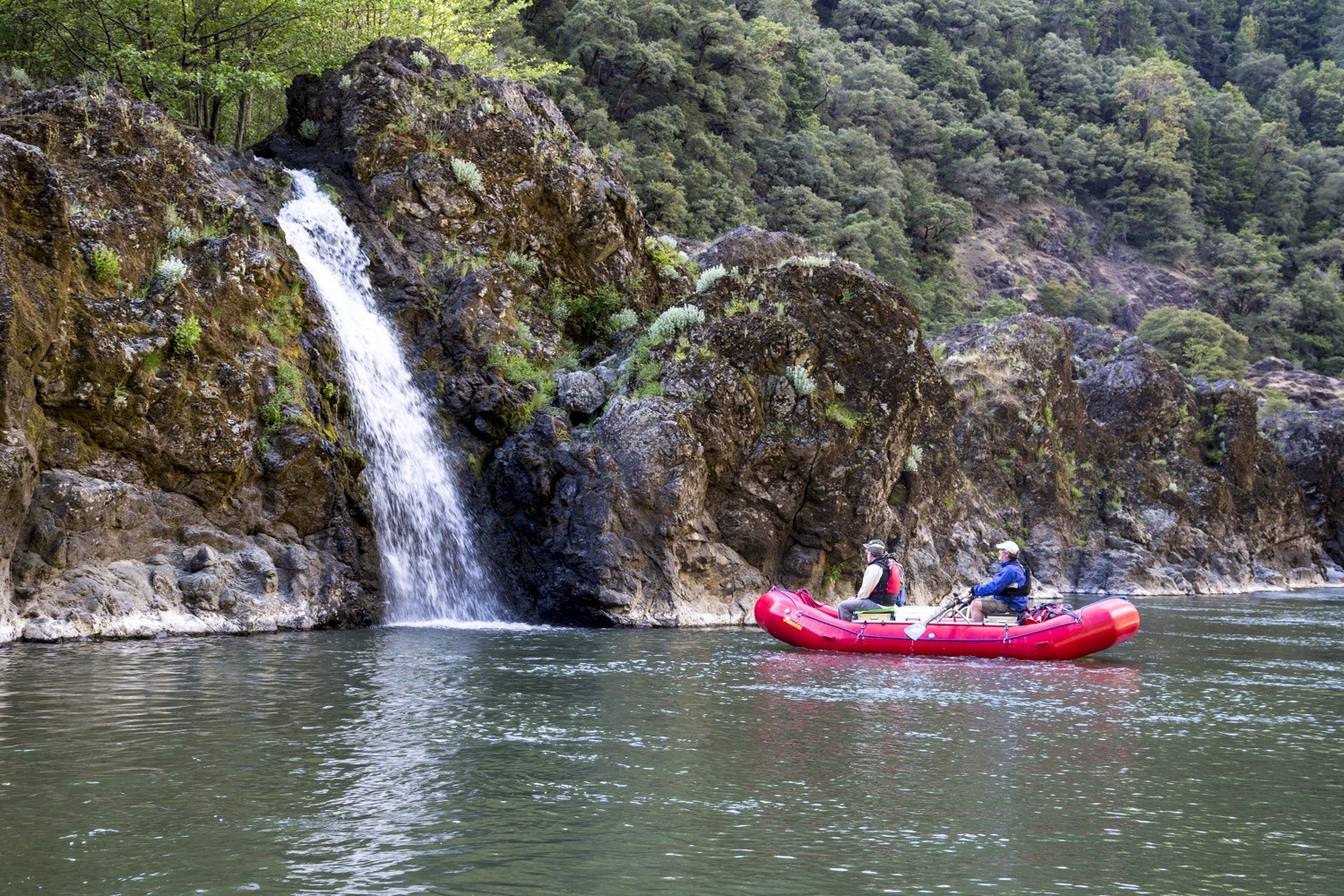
[838,540,905,622]
[965,541,1031,623]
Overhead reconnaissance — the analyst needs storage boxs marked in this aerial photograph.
[895,604,942,622]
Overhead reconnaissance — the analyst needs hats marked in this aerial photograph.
[867,543,884,557]
[996,541,1019,557]
[863,540,885,549]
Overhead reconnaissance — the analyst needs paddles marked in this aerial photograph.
[950,594,976,619]
[903,590,970,642]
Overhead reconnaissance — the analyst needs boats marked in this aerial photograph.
[754,586,1141,663]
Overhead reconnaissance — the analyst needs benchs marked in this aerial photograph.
[985,611,1019,625]
[857,610,894,621]
